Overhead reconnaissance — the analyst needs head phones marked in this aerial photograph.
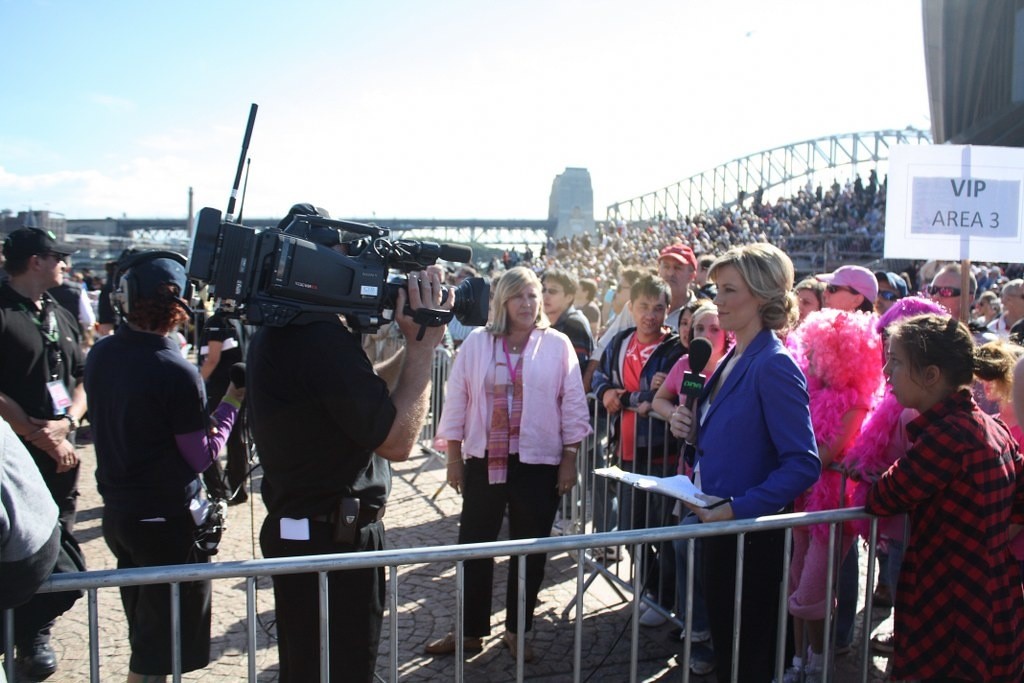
[108,250,200,315]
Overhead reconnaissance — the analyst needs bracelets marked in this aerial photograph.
[563,451,577,456]
[446,458,463,465]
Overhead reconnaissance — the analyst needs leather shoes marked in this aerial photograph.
[17,644,57,677]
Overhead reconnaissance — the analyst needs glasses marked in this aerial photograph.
[927,285,974,297]
[35,252,64,262]
[875,288,900,302]
[827,284,858,294]
[542,286,566,294]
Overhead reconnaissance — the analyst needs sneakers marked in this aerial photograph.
[640,607,674,626]
[638,592,655,615]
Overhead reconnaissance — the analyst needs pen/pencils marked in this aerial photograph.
[686,497,734,517]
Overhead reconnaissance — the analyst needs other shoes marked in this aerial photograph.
[772,664,809,683]
[586,546,623,561]
[691,638,714,674]
[804,660,836,683]
[871,591,895,617]
[552,519,574,534]
[874,631,895,653]
[425,630,483,654]
[679,624,711,642]
[835,642,852,656]
[504,631,532,661]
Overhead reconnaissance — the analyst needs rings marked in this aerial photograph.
[564,485,568,488]
[67,461,70,465]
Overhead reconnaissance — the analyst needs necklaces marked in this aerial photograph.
[512,344,517,350]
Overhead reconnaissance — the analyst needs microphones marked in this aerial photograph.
[439,242,472,263]
[229,362,247,387]
[678,337,713,447]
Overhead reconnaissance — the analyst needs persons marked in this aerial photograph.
[239,204,591,683]
[0,226,248,683]
[488,170,1024,683]
[864,312,1024,683]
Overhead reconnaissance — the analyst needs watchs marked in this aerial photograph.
[65,414,80,430]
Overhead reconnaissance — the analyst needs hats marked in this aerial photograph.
[2,227,72,259]
[873,271,908,298]
[121,257,195,316]
[815,264,878,303]
[658,244,698,270]
